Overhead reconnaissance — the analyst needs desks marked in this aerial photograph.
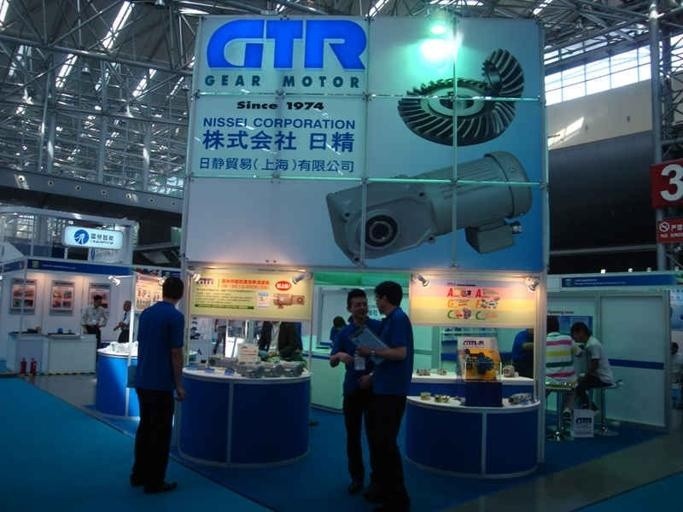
[95,344,140,420]
[406,394,541,480]
[179,358,311,468]
[411,370,534,399]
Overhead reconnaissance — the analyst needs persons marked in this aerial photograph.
[81,295,108,348]
[667,341,683,388]
[278,322,319,427]
[330,281,414,510]
[190,327,200,339]
[114,300,131,342]
[213,319,226,356]
[512,314,615,422]
[257,321,273,351]
[130,277,187,495]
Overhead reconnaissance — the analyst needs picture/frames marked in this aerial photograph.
[51,281,75,312]
[88,283,111,312]
[10,279,37,312]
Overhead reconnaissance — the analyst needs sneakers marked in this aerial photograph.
[347,479,410,511]
[144,482,175,493]
[130,474,143,486]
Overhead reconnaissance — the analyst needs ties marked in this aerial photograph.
[124,313,126,322]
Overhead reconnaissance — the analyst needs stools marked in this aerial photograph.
[594,379,624,438]
[545,382,580,445]
